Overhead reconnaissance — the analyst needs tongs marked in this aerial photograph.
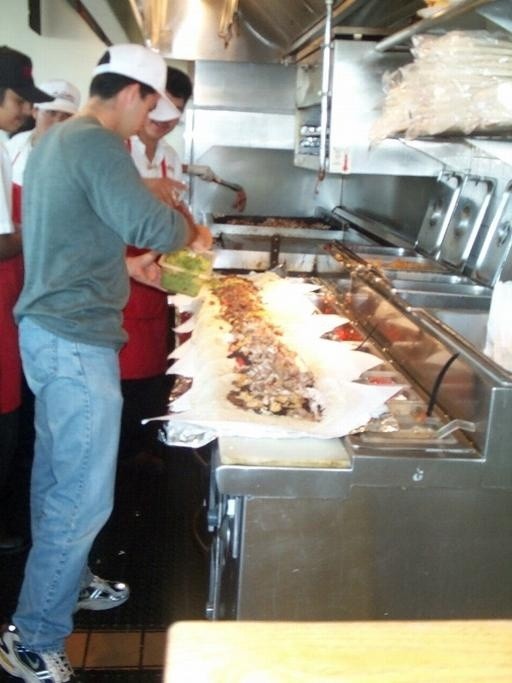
[211,175,242,191]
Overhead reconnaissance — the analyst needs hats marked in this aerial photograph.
[34,80,82,117]
[1,45,55,102]
[90,41,182,123]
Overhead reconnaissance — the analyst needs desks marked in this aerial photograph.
[163,616,512,683]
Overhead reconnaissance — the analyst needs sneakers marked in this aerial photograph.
[74,570,130,613]
[0,623,78,683]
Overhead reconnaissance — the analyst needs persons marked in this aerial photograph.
[1,35,222,683]
